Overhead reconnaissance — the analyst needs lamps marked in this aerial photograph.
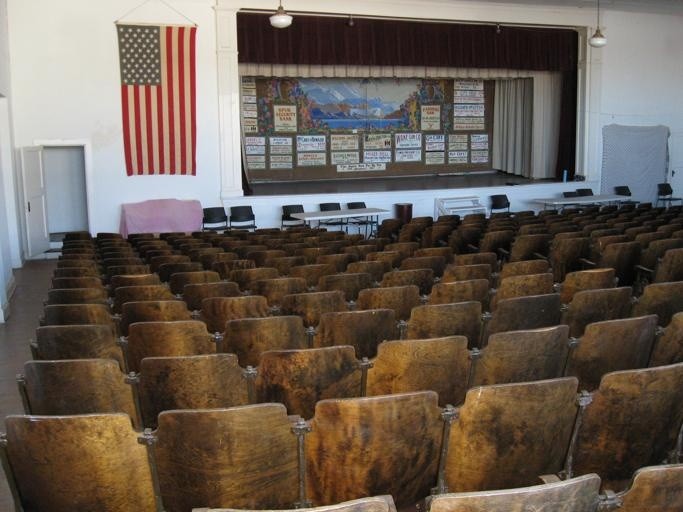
[268,1,293,30]
[588,1,606,49]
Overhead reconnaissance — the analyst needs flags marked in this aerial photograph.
[116,24,198,178]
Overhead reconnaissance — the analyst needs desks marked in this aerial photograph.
[534,194,632,211]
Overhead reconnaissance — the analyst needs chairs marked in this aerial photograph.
[655,184,682,208]
[563,190,579,209]
[614,185,631,197]
[577,190,601,212]
[489,195,511,213]
